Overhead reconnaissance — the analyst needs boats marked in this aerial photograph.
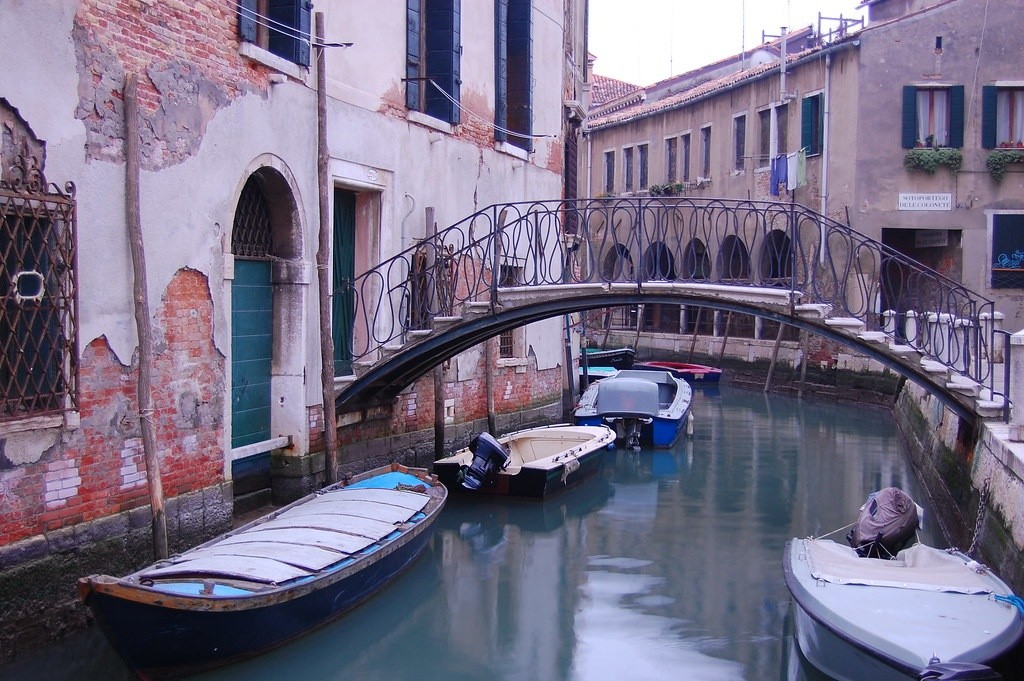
[429,423,618,510]
[633,362,721,386]
[72,461,448,680]
[569,365,694,451]
[783,485,1024,681]
[578,347,636,371]
[577,365,618,398]
